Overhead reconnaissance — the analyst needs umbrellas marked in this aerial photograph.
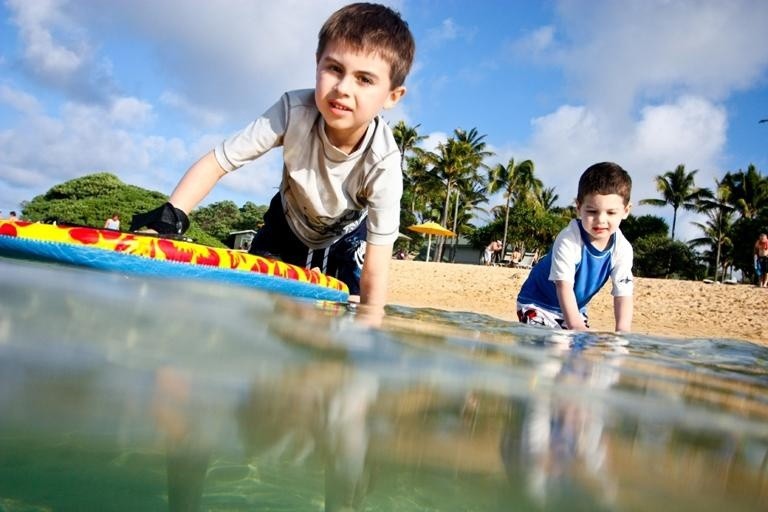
[406,221,458,262]
[396,232,413,242]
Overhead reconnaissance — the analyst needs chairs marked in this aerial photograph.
[492,250,537,269]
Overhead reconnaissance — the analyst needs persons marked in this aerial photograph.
[103,213,120,230]
[515,161,636,341]
[508,247,522,268]
[482,239,502,266]
[752,232,768,288]
[8,212,17,222]
[152,327,374,512]
[396,244,408,260]
[532,246,540,267]
[503,331,626,511]
[129,0,414,329]
